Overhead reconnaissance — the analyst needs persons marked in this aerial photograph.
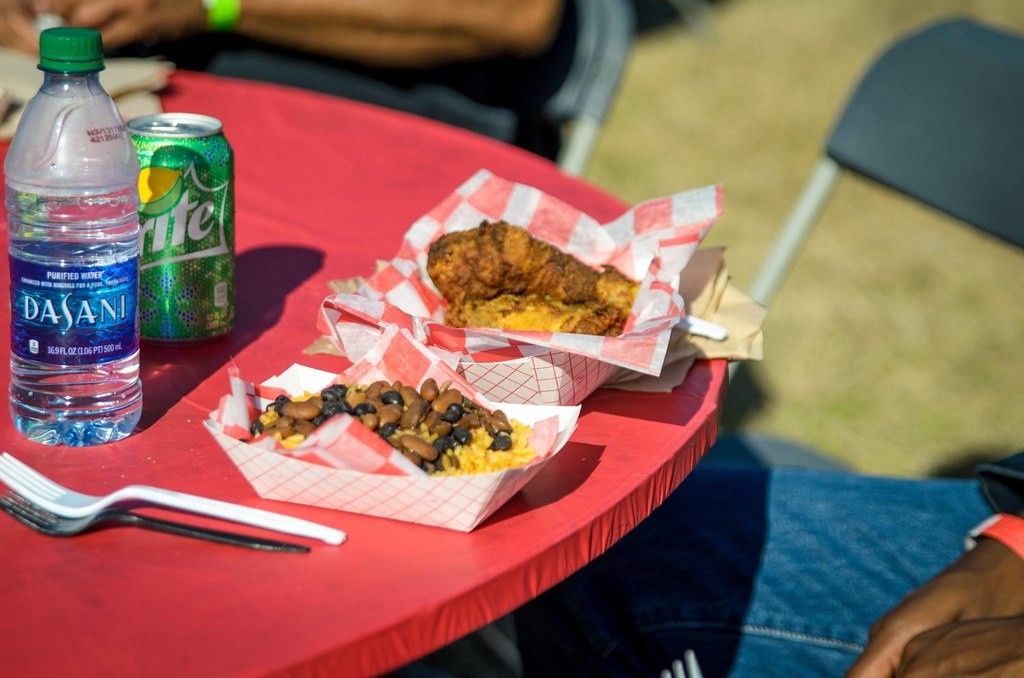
[0,0,571,149]
[515,448,1023,678]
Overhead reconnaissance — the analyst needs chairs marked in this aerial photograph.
[112,0,634,187]
[724,12,1022,400]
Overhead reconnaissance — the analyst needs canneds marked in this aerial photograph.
[124,113,237,339]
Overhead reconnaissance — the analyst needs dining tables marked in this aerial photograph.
[0,41,728,678]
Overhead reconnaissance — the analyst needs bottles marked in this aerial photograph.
[2,25,144,446]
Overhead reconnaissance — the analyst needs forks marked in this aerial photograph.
[0,449,347,546]
[0,489,311,570]
